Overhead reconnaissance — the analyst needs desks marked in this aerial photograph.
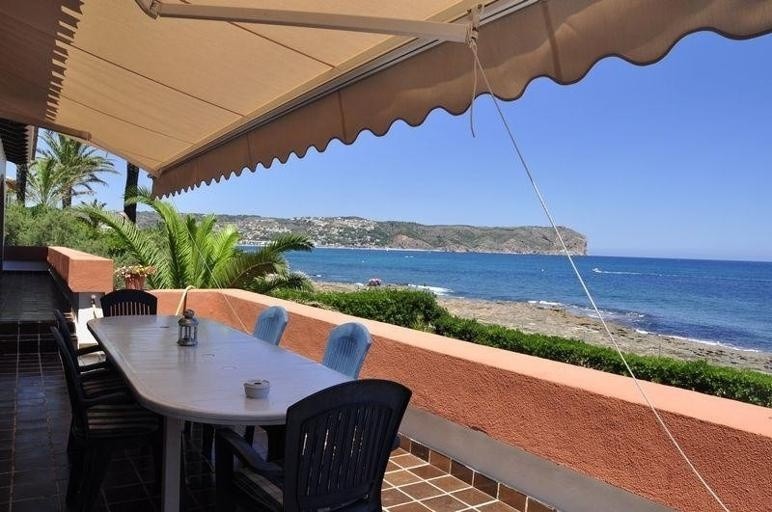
[87,314,355,512]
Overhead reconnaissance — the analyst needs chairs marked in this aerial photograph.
[184,308,289,461]
[242,322,374,470]
[216,378,414,511]
[100,287,157,316]
[50,324,184,510]
[53,308,132,394]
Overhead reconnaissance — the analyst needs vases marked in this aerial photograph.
[125,275,146,291]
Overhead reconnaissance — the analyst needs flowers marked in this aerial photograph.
[112,264,156,279]
